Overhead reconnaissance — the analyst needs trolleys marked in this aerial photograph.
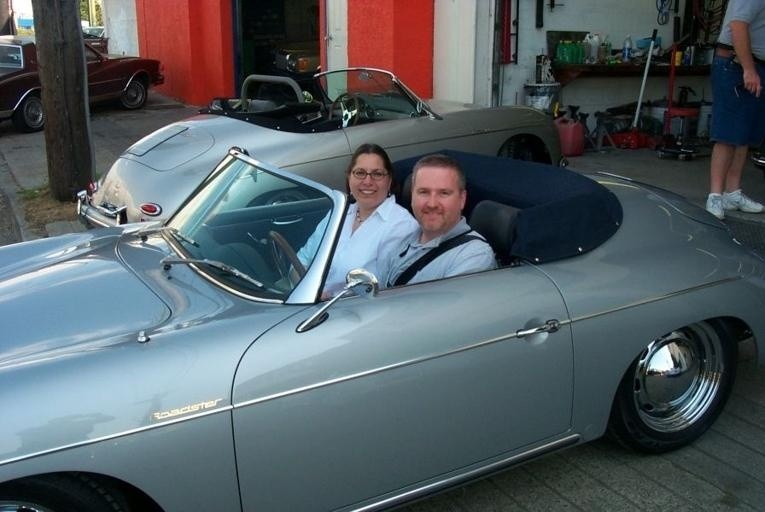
[653,16,713,162]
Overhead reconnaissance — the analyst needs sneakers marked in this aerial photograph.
[723,189,764,213]
[707,195,725,220]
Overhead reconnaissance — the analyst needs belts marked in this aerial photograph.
[715,48,735,58]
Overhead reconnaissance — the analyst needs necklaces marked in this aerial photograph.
[355,208,365,226]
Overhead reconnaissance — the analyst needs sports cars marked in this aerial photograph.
[72,54,571,239]
[1,148,765,512]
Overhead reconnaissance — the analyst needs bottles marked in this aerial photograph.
[557,33,622,65]
[623,33,633,65]
[684,47,692,65]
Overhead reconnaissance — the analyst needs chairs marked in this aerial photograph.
[470,201,522,254]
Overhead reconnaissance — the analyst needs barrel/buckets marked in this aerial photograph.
[553,117,584,157]
[652,98,673,121]
[584,32,600,59]
[696,105,713,139]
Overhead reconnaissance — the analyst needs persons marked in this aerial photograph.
[705,1,764,221]
[288,143,420,302]
[319,153,499,303]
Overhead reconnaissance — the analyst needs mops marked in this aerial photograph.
[617,29,658,151]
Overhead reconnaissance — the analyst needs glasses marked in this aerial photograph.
[349,168,386,180]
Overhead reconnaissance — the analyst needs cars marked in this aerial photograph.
[244,11,321,77]
[82,26,106,52]
[0,33,164,132]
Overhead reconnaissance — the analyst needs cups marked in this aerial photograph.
[672,52,683,65]
